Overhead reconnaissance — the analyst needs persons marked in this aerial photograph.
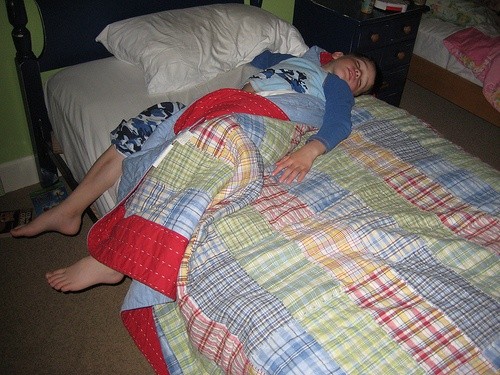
[9,48,383,293]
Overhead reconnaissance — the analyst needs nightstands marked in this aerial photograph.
[293,2,426,107]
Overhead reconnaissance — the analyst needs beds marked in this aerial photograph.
[5,1,499,375]
[407,0,500,128]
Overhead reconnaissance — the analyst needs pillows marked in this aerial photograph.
[94,3,310,95]
